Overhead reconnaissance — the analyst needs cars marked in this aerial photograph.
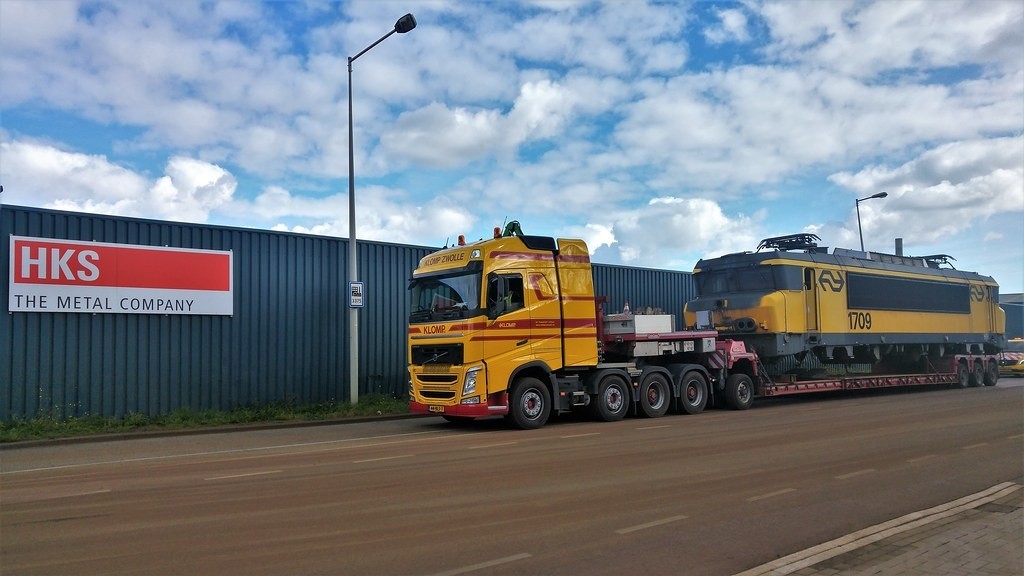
[996,337,1024,377]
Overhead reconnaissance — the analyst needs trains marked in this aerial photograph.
[683,233,1007,375]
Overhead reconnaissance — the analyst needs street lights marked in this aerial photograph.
[340,12,417,407]
[856,192,888,250]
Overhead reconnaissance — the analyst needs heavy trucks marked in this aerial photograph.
[408,215,1008,431]
